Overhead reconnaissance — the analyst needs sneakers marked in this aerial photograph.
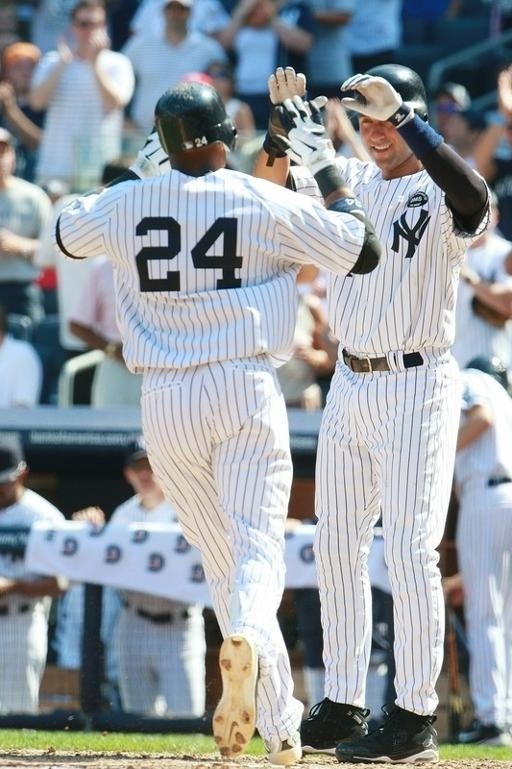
[213,635,257,757]
[339,708,440,764]
[450,722,511,748]
[301,697,369,754]
[265,728,301,765]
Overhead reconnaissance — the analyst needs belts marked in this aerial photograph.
[2,603,30,617]
[342,350,419,373]
[137,609,171,627]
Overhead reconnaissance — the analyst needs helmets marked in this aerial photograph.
[156,82,238,155]
[350,65,429,130]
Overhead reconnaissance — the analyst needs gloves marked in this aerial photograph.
[274,95,348,197]
[342,74,446,161]
[263,67,328,157]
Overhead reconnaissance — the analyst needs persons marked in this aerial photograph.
[0,367,512,746]
[1,1,512,412]
[250,65,490,765]
[52,80,381,767]
